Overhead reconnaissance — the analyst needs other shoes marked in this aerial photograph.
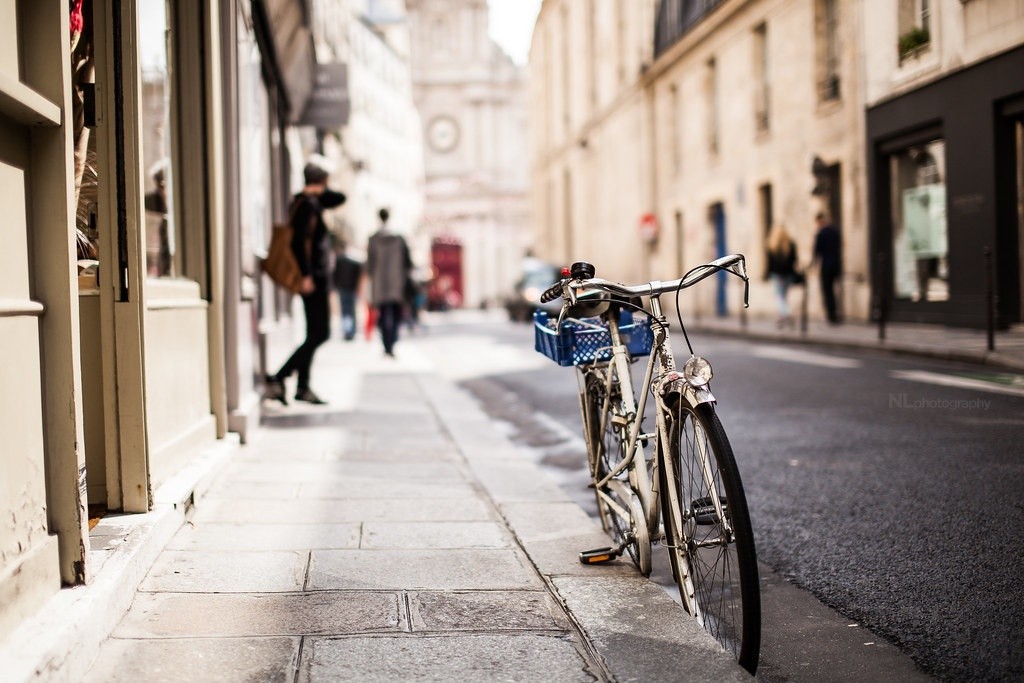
[295,389,326,404]
[270,376,288,405]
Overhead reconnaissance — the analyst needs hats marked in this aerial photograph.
[304,163,327,185]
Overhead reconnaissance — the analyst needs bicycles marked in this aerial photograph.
[532,252,763,677]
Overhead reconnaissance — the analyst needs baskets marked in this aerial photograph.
[534,308,653,367]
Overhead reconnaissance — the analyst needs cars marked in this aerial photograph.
[505,264,578,325]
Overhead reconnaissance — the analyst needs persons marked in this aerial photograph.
[807,210,844,323]
[264,159,346,407]
[331,244,361,338]
[760,223,799,323]
[367,206,425,359]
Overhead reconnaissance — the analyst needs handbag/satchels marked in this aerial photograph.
[263,199,315,292]
